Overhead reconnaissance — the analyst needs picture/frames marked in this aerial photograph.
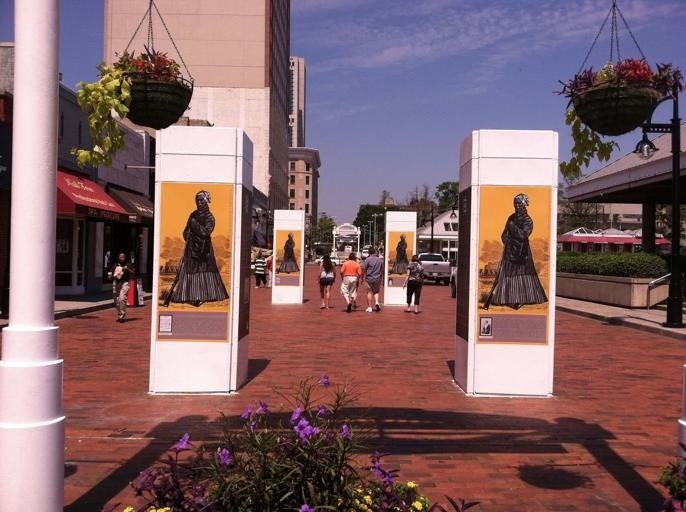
[158,314,172,334]
[480,317,492,336]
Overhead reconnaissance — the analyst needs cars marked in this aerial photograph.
[360,239,384,260]
[450,266,457,298]
[314,256,345,266]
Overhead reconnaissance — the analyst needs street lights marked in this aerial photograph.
[372,213,383,250]
[450,198,459,220]
[256,206,271,250]
[632,72,685,329]
[362,220,373,246]
[420,205,434,253]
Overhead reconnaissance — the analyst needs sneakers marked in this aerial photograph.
[365,308,372,312]
[375,303,381,311]
[319,304,326,309]
[347,303,352,312]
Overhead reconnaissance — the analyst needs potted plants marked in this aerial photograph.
[70,0,193,170]
[552,0,683,181]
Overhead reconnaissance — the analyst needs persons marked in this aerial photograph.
[252,249,267,291]
[107,252,136,323]
[339,252,360,313]
[159,189,230,307]
[314,255,338,311]
[265,255,274,289]
[345,256,365,308]
[402,254,427,316]
[276,232,300,276]
[486,191,549,311]
[362,247,385,312]
[389,232,411,278]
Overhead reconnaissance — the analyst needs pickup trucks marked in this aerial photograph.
[415,252,451,286]
